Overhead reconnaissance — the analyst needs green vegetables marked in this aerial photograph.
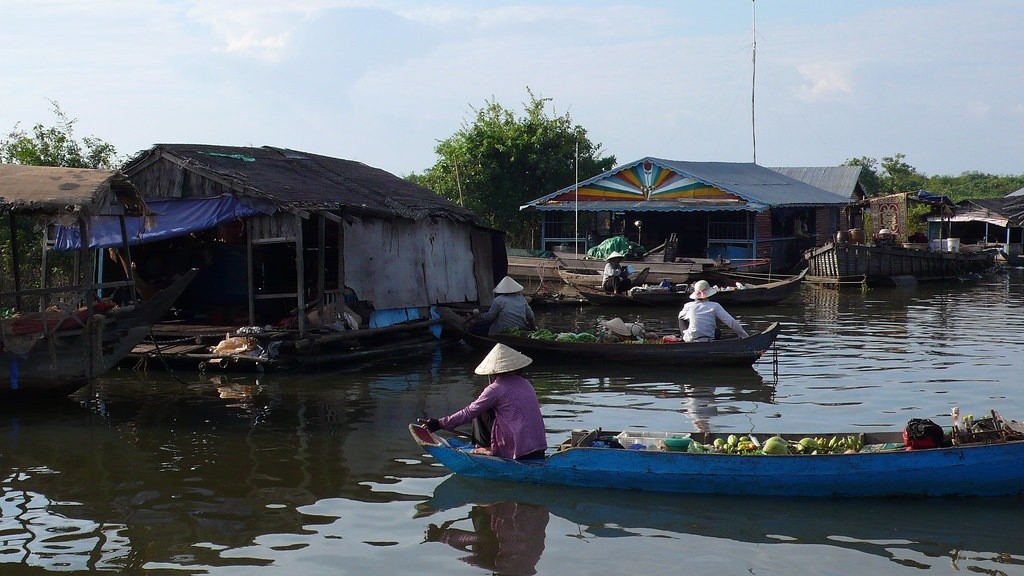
[501,324,596,342]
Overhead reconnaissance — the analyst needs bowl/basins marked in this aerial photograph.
[879,229,891,234]
[902,242,929,250]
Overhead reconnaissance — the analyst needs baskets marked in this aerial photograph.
[617,430,691,450]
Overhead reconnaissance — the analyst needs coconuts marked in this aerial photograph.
[761,436,818,455]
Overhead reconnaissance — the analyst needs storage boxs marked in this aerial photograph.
[617,430,692,451]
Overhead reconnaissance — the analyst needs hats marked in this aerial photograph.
[606,251,624,261]
[690,280,716,299]
[475,343,532,375]
[493,276,523,293]
[606,318,631,336]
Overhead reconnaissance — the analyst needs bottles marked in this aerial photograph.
[953,414,974,435]
[663,279,668,289]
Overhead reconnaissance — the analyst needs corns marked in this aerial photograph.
[713,434,752,455]
[814,435,857,452]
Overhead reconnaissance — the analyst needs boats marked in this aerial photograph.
[434,305,780,371]
[804,227,999,285]
[505,246,716,285]
[409,423,1024,503]
[578,266,810,303]
[0,162,199,405]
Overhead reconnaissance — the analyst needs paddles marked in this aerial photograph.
[462,309,486,325]
[567,279,657,307]
[417,418,473,440]
[643,239,668,257]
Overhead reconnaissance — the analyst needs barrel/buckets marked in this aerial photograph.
[665,435,691,451]
[849,229,864,244]
[947,238,960,253]
[837,231,852,243]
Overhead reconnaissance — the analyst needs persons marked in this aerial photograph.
[473,276,538,339]
[602,251,632,295]
[421,343,547,463]
[433,501,549,576]
[678,280,749,343]
[908,227,929,243]
[968,231,999,244]
[183,247,229,320]
